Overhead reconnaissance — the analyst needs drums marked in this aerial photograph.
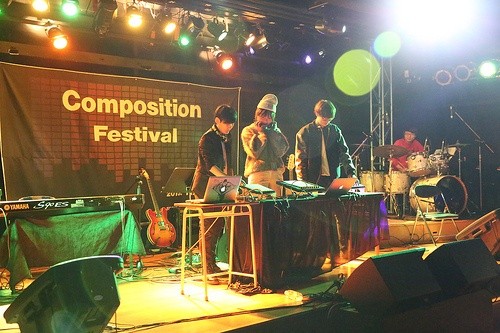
[360,170,384,192]
[383,170,410,195]
[408,174,468,215]
[428,153,451,174]
[405,151,434,178]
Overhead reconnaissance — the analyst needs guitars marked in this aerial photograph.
[285,153,295,180]
[138,167,177,248]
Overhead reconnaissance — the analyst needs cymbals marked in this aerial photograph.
[347,144,371,149]
[447,143,472,147]
[372,144,409,158]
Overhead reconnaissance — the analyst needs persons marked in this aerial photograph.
[392,126,424,171]
[295,100,358,264]
[241,94,289,197]
[190,104,238,284]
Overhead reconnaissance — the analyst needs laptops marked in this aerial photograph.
[317,177,357,197]
[185,176,242,204]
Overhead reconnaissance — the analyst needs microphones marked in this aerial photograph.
[442,140,444,152]
[386,112,388,124]
[449,106,453,118]
[424,139,427,151]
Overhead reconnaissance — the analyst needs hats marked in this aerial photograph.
[256,93,278,113]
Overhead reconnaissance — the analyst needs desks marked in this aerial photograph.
[198,192,390,289]
[0,209,147,295]
[174,202,259,301]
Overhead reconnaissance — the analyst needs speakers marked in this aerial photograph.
[3,255,125,333]
[338,208,500,320]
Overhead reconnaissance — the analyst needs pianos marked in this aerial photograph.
[0,193,145,282]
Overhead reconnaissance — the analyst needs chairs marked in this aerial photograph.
[410,185,459,247]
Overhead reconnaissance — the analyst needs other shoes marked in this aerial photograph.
[202,264,218,284]
[211,262,226,278]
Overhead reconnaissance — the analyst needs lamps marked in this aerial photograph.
[93,0,270,69]
[45,27,68,49]
[314,17,346,37]
[435,59,500,86]
